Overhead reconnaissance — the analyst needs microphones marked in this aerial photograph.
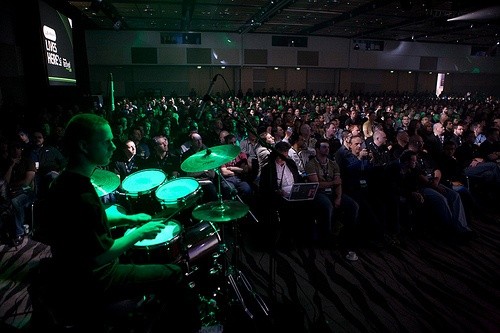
[282,182,320,202]
[195,77,217,123]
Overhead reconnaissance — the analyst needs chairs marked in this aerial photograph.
[6,172,38,237]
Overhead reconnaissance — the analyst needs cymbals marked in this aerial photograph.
[191,197,250,223]
[179,143,242,174]
[90,167,122,198]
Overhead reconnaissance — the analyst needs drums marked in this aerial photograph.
[121,168,168,216]
[124,217,184,264]
[102,204,129,228]
[183,221,223,269]
[155,177,204,222]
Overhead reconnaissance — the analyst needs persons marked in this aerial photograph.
[0,86,500,238]
[30,113,186,333]
